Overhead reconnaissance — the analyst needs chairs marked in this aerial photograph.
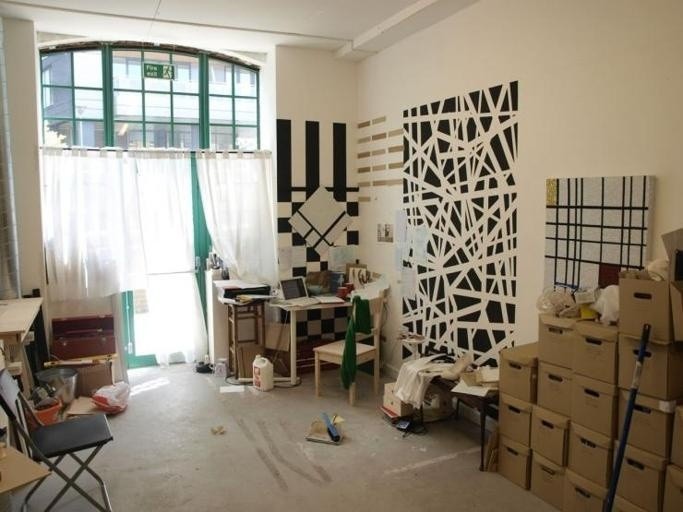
[0,369,114,512]
[313,288,384,407]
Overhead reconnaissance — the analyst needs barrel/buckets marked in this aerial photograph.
[253,354,274,391]
[41,359,77,404]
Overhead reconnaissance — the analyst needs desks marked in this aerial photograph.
[270,296,353,386]
[0,297,44,397]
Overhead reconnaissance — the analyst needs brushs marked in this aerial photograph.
[321,412,340,442]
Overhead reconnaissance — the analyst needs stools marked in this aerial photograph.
[227,303,265,381]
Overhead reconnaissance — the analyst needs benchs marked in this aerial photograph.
[414,357,499,472]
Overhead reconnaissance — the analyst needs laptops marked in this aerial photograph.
[278,276,321,308]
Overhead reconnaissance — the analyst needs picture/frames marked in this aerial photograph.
[346,264,367,287]
[376,223,395,245]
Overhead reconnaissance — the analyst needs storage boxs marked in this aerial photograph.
[382,383,413,417]
[617,275,682,347]
[494,439,529,492]
[537,314,572,370]
[498,353,538,404]
[610,441,661,510]
[669,407,683,469]
[497,393,533,448]
[535,365,572,418]
[52,314,116,360]
[616,388,672,459]
[528,450,561,509]
[608,495,636,510]
[660,465,683,512]
[570,425,612,488]
[571,372,617,437]
[617,338,670,399]
[562,472,607,512]
[570,321,616,384]
[531,404,570,467]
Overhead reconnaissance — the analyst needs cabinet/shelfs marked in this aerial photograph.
[204,269,224,365]
